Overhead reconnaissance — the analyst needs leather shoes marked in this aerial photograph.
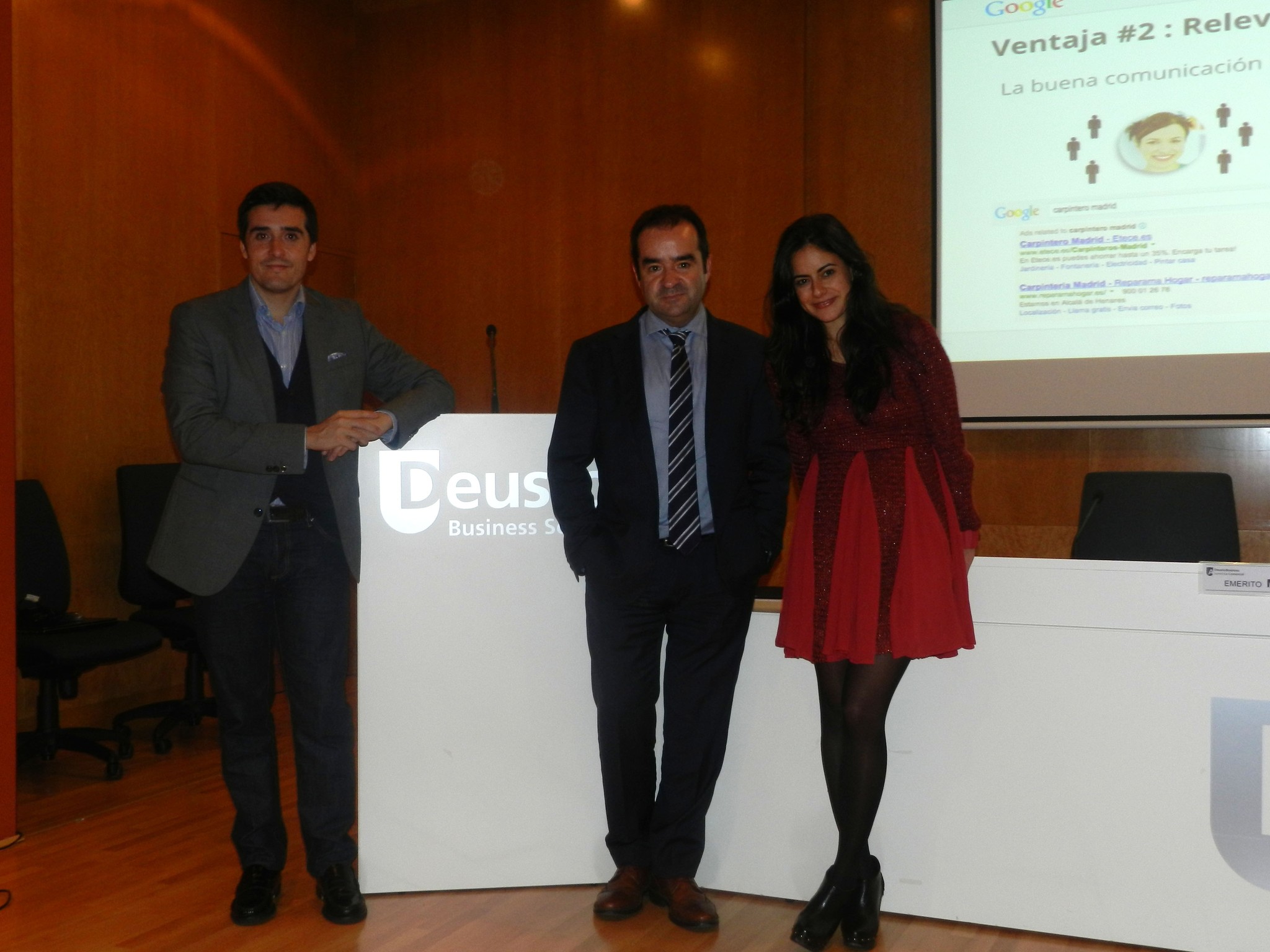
[651,876,719,928]
[313,864,368,924]
[228,864,283,925]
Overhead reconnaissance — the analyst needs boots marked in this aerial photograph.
[840,855,885,951]
[790,866,861,951]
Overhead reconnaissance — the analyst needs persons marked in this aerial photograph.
[144,178,463,927]
[545,200,786,932]
[760,210,984,950]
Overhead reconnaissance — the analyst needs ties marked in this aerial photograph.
[658,328,703,559]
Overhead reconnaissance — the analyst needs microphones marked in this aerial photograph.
[486,324,496,336]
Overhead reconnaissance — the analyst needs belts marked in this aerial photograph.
[592,865,647,918]
[264,505,319,526]
[657,534,717,551]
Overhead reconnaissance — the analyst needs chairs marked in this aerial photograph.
[15,477,160,784]
[1069,471,1239,567]
[115,461,219,758]
